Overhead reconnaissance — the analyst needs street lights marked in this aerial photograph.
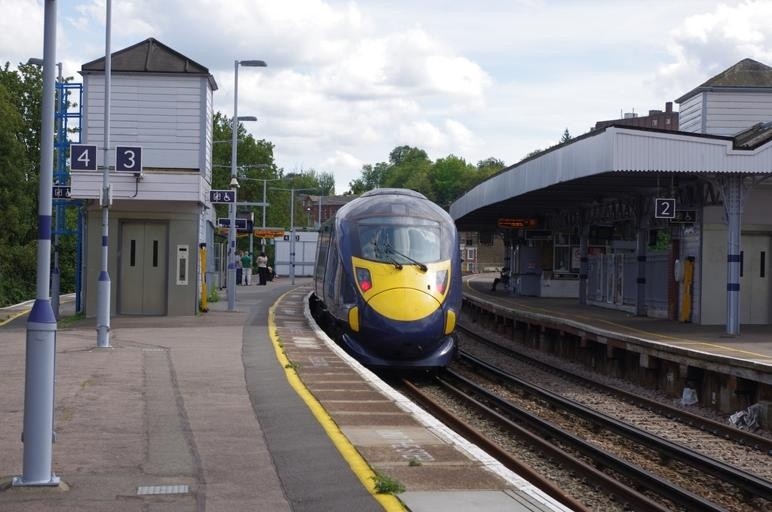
[213,60,310,312]
[26,58,62,321]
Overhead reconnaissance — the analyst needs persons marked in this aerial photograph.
[236,248,245,285]
[242,251,254,285]
[491,268,509,291]
[256,251,268,285]
[266,264,279,281]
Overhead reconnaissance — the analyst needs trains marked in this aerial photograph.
[313,188,463,367]
